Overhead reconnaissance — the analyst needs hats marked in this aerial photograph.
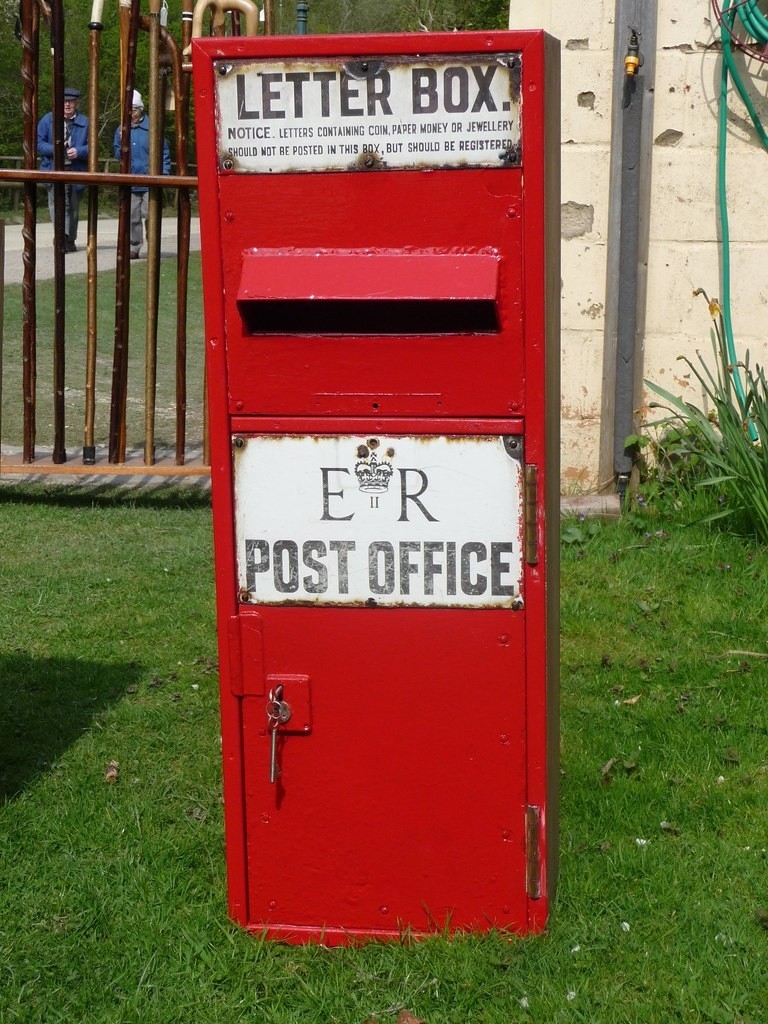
[64,88,80,100]
[132,90,144,110]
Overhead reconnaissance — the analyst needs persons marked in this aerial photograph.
[113,91,170,258]
[37,88,89,251]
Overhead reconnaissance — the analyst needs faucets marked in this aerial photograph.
[624,26,642,77]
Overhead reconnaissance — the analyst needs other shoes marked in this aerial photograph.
[64,241,76,252]
[130,252,139,259]
